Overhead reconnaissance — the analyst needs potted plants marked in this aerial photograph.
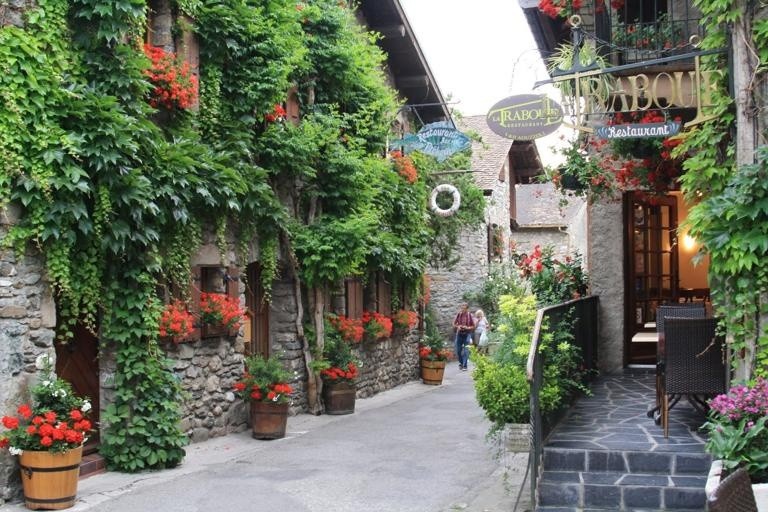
[461,345,566,453]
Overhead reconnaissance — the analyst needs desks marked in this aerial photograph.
[644,321,656,328]
[632,331,659,343]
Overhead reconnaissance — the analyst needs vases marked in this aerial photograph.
[251,401,288,440]
[420,360,446,385]
[19,444,83,510]
[324,382,356,414]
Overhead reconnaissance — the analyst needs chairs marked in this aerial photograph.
[647,288,728,439]
[707,466,760,512]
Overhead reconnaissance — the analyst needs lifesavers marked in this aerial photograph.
[430,183,460,218]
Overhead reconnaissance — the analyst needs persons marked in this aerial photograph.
[473,308,490,355]
[451,301,476,371]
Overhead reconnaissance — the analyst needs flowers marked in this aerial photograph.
[698,373,768,476]
[331,309,418,344]
[155,281,252,345]
[507,240,588,304]
[390,150,419,184]
[142,43,199,112]
[252,102,289,144]
[231,349,294,404]
[308,356,359,384]
[0,356,99,452]
[420,334,454,362]
[528,0,688,214]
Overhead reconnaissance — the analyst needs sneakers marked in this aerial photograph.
[459,364,467,371]
